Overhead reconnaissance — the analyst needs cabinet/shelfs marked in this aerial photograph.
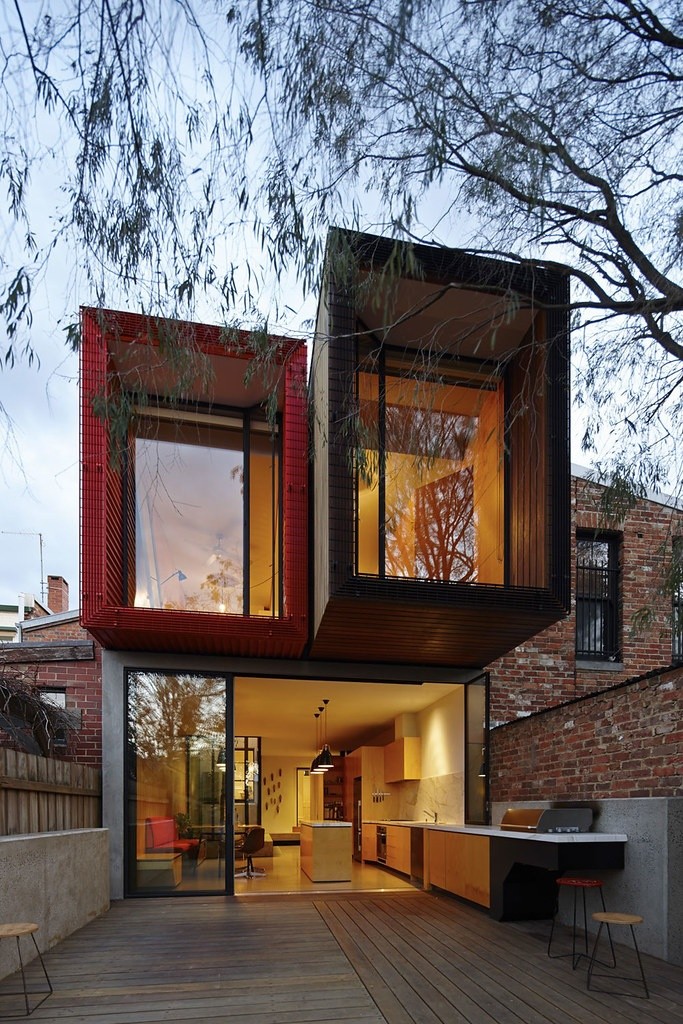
[346,746,399,862]
[429,829,625,922]
[316,764,345,822]
[363,824,423,889]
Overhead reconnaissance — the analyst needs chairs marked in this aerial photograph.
[234,828,266,879]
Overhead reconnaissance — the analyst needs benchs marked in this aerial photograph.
[145,817,207,874]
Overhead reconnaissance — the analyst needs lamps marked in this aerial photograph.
[309,714,325,775]
[216,748,227,766]
[161,570,188,587]
[222,764,237,772]
[317,700,334,768]
[313,707,329,773]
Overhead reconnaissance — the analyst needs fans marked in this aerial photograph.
[201,533,240,566]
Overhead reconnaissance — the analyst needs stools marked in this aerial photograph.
[587,912,649,1000]
[547,878,616,970]
[0,923,53,1018]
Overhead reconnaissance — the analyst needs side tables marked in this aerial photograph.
[137,853,182,887]
